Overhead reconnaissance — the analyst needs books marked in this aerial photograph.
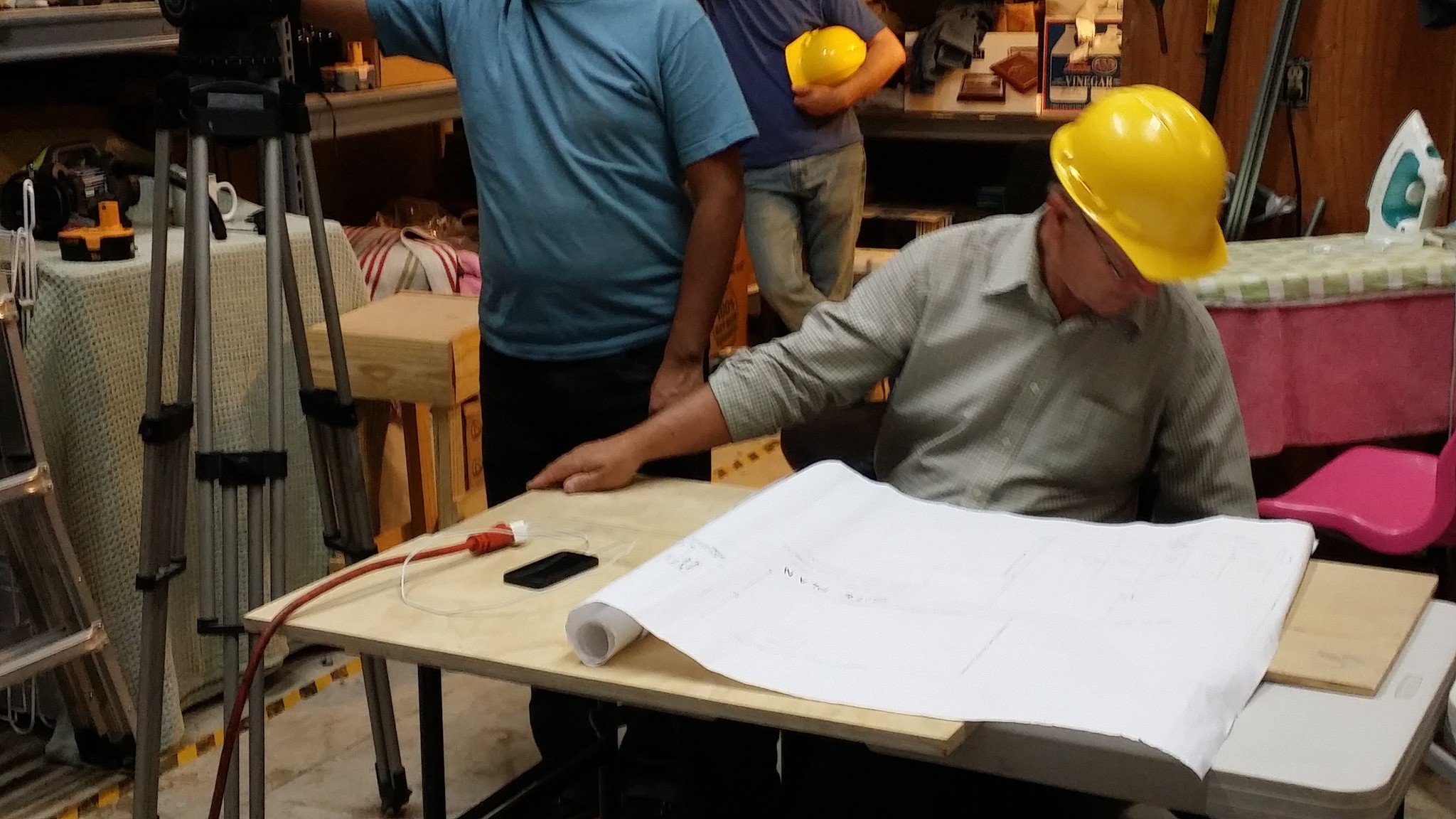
[1264,540,1441,696]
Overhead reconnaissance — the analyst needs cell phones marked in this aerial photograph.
[503,549,599,590]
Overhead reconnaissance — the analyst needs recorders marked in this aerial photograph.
[1,139,141,241]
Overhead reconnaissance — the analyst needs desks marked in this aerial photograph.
[1199,222,1454,459]
[249,473,1454,819]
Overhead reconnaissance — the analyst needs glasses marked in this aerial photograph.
[1077,208,1145,285]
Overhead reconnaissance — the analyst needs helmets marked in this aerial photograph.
[784,25,868,98]
[1047,80,1231,283]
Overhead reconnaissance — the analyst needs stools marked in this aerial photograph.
[304,290,490,539]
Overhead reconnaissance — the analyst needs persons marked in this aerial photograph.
[307,1,758,765]
[698,1,906,405]
[522,83,1264,536]
